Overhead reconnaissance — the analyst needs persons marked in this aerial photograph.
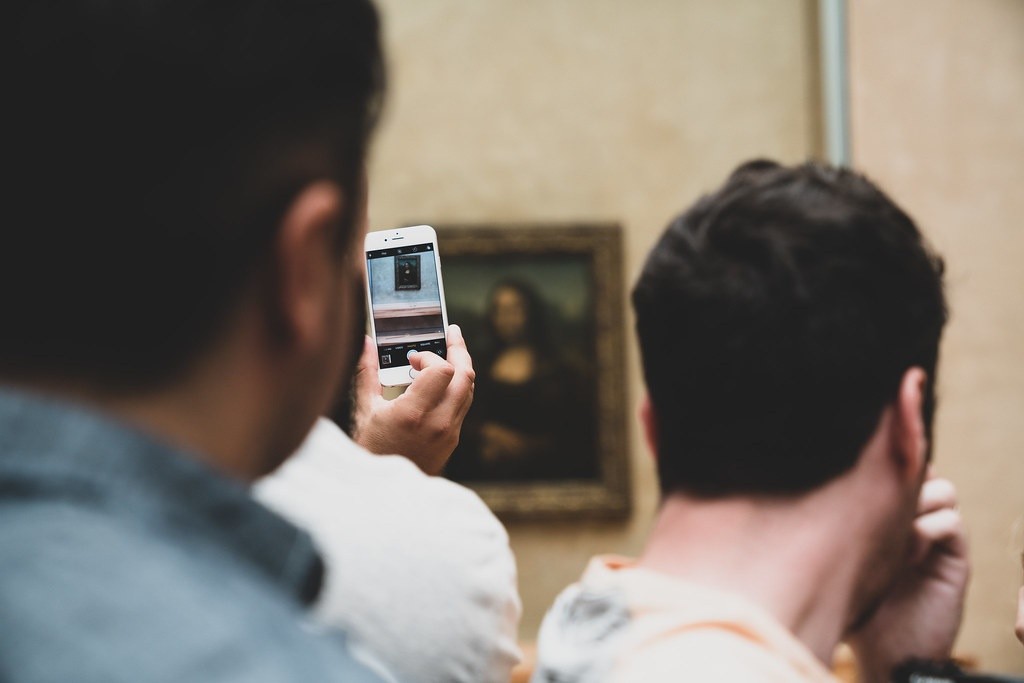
[0,0,1024,683]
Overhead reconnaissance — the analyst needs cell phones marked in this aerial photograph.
[363,225,449,386]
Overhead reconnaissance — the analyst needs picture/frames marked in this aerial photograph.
[394,255,421,290]
[377,223,636,520]
